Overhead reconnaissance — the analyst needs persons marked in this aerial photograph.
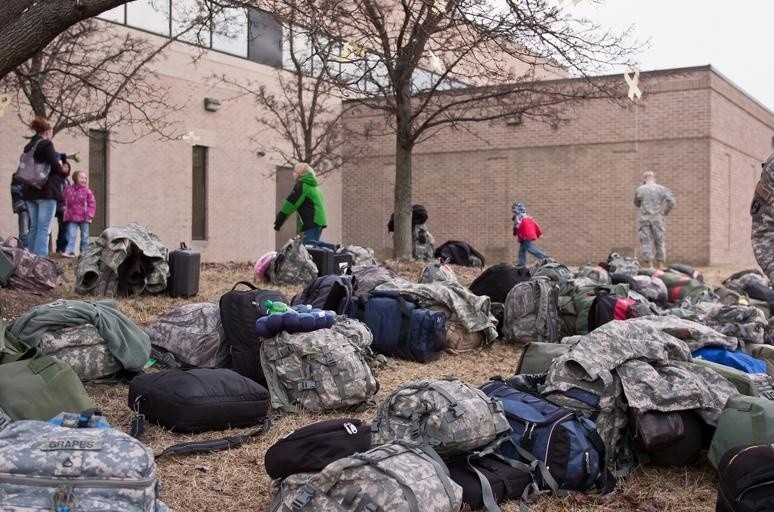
[633,171,675,271]
[750,150,773,289]
[58,171,95,256]
[54,159,71,253]
[510,202,549,269]
[21,120,64,259]
[49,151,81,162]
[272,162,327,245]
[10,152,32,241]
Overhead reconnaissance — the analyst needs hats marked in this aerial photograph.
[643,171,654,178]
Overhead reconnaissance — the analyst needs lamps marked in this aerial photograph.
[205,98,220,112]
[505,114,521,124]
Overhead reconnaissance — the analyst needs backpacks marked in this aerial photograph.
[388,205,427,232]
[412,223,434,260]
[0,232,774,512]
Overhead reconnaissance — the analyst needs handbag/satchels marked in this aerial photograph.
[13,139,51,191]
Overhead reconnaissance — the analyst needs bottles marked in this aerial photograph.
[264,300,301,316]
